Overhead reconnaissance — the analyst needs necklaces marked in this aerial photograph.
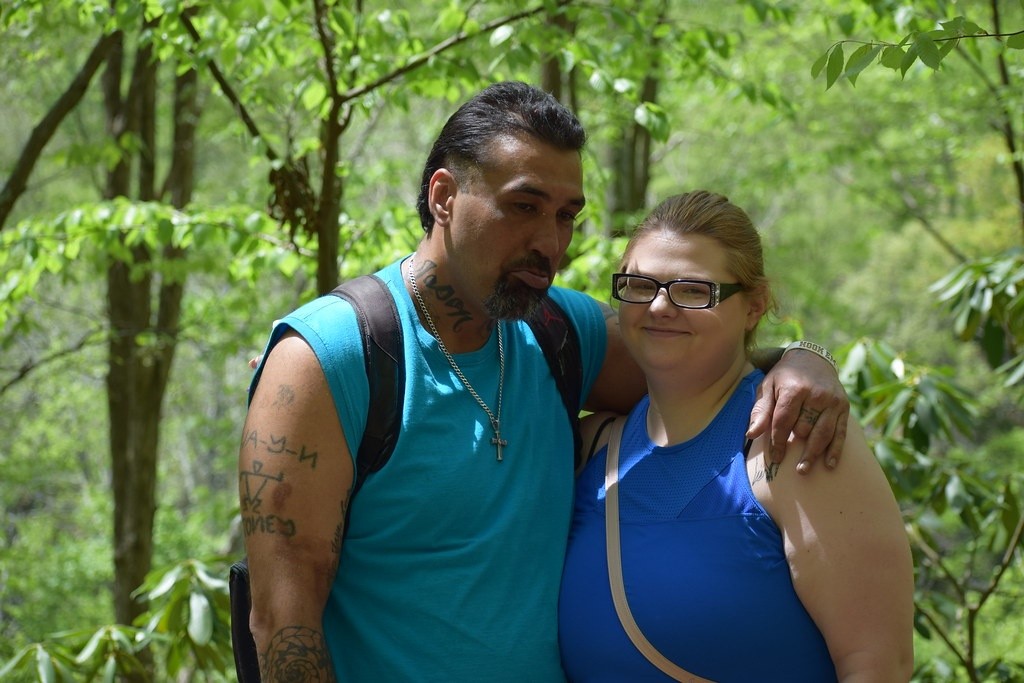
[409,256,507,461]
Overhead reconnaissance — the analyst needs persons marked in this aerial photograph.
[237,79,851,683]
[558,190,915,683]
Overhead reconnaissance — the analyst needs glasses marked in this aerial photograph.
[611,273,742,309]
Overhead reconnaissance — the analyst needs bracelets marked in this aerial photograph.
[781,341,839,376]
